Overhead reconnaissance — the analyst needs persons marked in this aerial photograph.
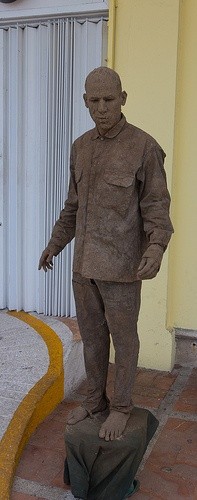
[37,65,176,441]
